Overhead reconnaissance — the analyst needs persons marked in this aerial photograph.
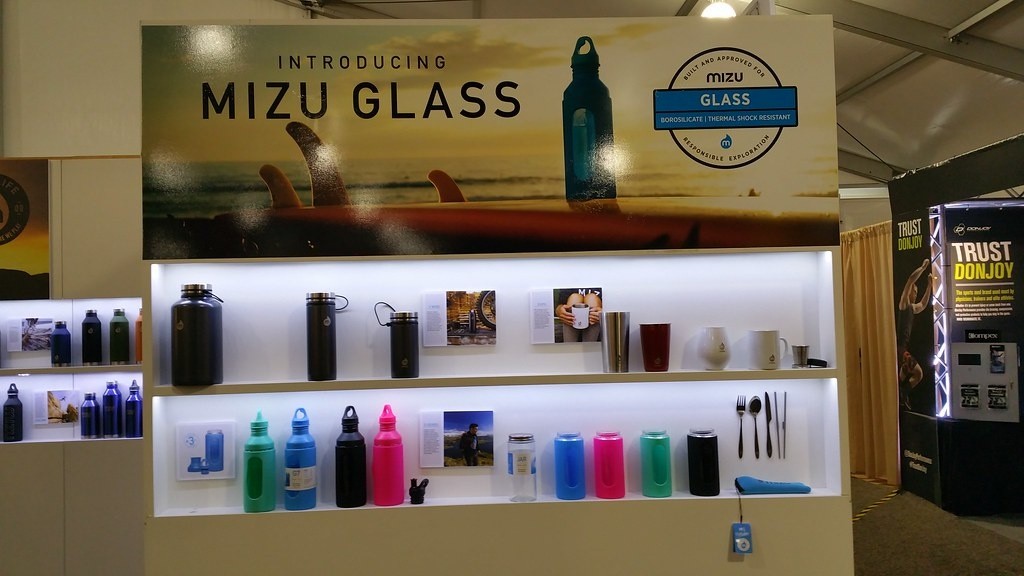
[554,288,603,342]
[460,423,480,466]
[991,350,1003,365]
[896,258,933,410]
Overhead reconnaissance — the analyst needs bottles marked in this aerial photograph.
[554,431,586,499]
[593,428,626,499]
[508,433,542,502]
[205,430,225,472]
[242,405,406,513]
[469,309,477,332]
[171,283,421,385]
[686,428,720,496]
[563,35,616,201]
[639,428,673,497]
[1,306,144,442]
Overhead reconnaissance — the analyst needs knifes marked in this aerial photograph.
[765,391,772,457]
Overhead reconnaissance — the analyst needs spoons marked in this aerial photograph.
[748,396,762,459]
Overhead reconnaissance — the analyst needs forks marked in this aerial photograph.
[736,395,746,458]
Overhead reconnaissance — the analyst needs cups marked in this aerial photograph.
[571,304,592,329]
[639,323,672,372]
[747,328,789,370]
[599,311,630,373]
[791,344,810,368]
[696,326,732,371]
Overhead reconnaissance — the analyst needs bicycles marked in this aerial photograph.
[476,291,496,331]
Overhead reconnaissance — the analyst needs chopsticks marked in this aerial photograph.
[774,392,787,460]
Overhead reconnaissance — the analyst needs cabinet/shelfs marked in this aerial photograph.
[140,248,856,576]
[0,156,142,576]
